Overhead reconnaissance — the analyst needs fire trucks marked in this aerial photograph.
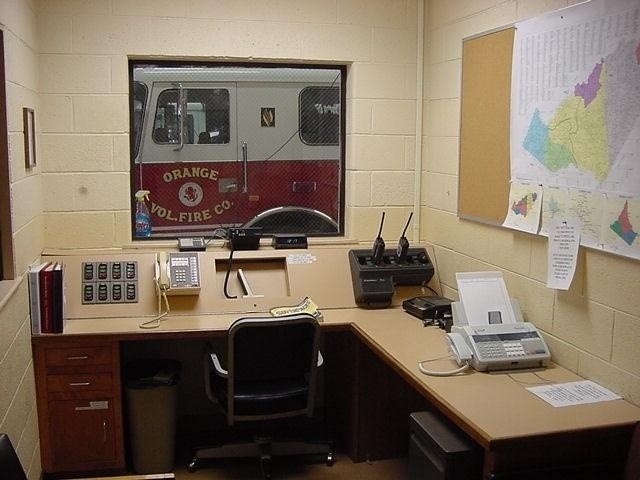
[132,66,340,240]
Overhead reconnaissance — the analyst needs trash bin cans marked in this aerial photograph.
[124,361,185,473]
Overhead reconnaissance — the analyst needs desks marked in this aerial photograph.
[31,300,640,480]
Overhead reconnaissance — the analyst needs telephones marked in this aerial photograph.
[446,331,471,364]
[154,251,201,297]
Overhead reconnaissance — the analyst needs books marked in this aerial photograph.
[268,295,326,324]
[27,261,49,336]
[51,261,68,334]
[40,260,56,334]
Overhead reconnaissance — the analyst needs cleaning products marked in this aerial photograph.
[135,189,150,237]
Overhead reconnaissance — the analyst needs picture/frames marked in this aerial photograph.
[23,107,36,168]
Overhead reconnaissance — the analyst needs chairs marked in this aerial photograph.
[189,313,335,480]
[0,433,176,480]
